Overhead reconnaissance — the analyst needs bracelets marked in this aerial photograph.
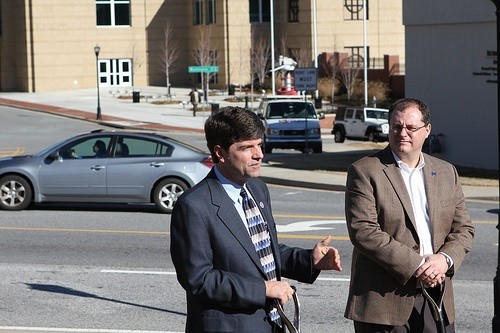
[446,257,451,268]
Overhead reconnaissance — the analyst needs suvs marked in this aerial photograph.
[330,107,390,143]
[255,99,323,154]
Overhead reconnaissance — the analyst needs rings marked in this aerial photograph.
[432,272,436,277]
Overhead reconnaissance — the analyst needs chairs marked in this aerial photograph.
[115,143,129,157]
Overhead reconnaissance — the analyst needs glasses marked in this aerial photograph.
[390,124,428,133]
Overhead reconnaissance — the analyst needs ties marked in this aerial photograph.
[240,187,283,329]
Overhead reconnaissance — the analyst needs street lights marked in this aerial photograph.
[94,44,102,120]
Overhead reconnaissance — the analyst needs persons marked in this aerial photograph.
[344,98,475,333]
[72,140,106,160]
[170,106,343,333]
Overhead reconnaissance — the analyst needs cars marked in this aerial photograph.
[0,131,215,214]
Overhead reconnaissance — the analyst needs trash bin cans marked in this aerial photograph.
[316,97,322,109]
[133,92,140,103]
[229,85,235,95]
